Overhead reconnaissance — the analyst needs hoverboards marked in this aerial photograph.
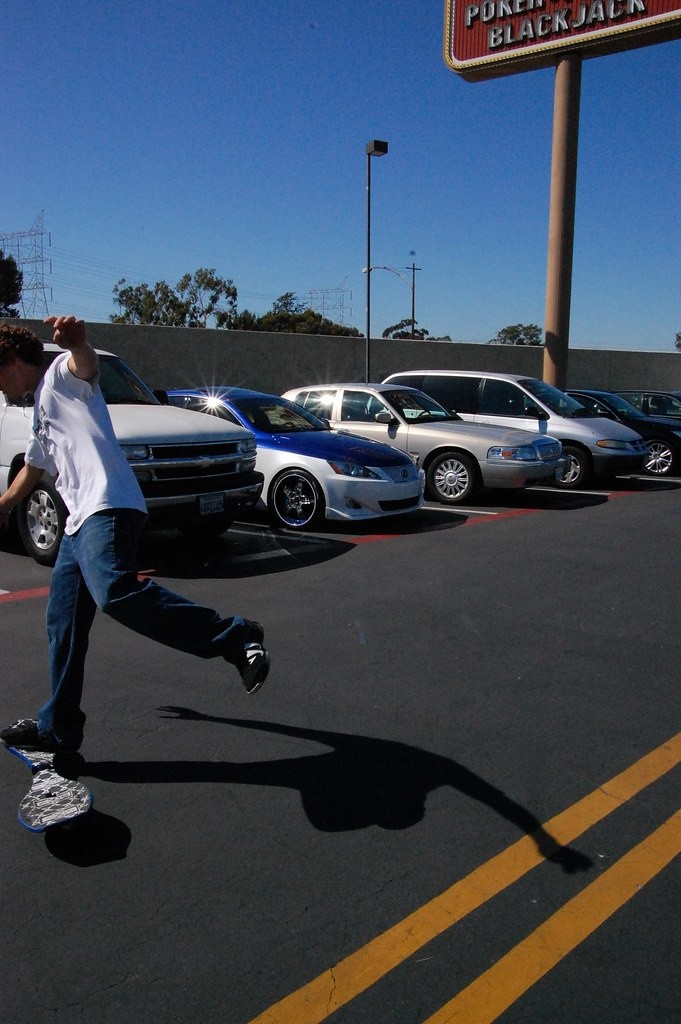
[2,719,93,848]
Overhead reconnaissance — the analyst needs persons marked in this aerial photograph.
[0,316,270,744]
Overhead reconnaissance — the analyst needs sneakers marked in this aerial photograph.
[0,714,87,752]
[231,617,270,694]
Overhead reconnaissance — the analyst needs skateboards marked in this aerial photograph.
[5,714,96,833]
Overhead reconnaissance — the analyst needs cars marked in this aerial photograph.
[0,341,263,568]
[591,389,681,422]
[381,370,648,491]
[152,383,426,529]
[510,389,681,476]
[278,383,563,507]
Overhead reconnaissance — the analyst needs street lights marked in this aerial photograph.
[363,266,416,341]
[365,139,387,382]
